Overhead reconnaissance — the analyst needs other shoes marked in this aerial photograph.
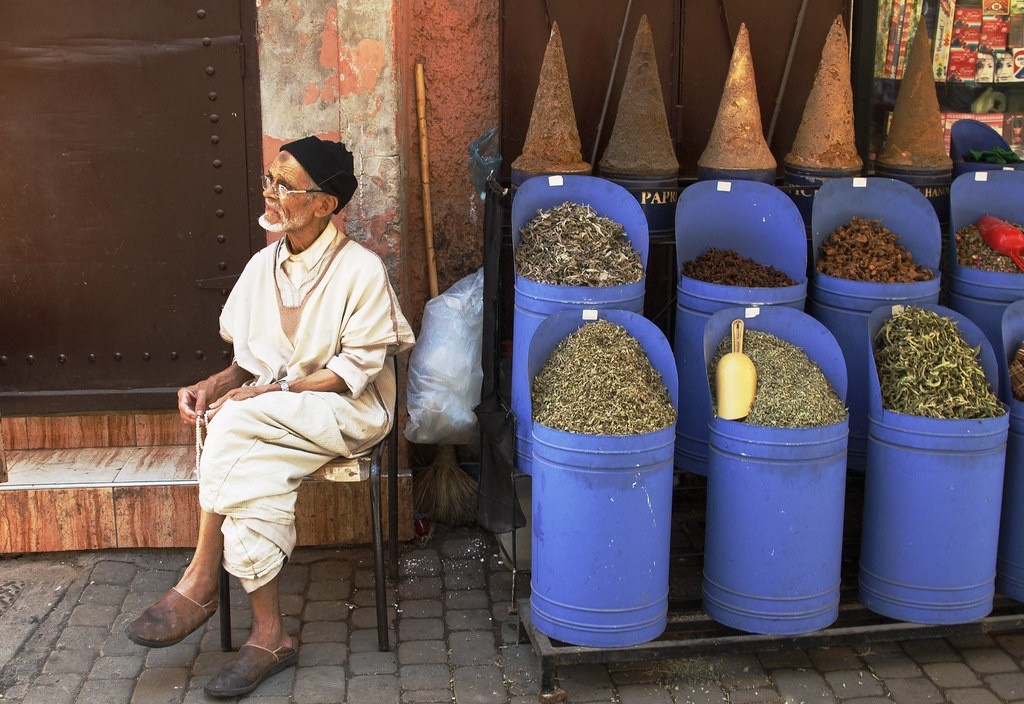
[204,644,298,697]
[124,587,219,648]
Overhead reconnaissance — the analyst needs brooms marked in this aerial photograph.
[410,57,478,527]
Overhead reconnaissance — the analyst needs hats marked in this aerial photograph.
[279,136,357,215]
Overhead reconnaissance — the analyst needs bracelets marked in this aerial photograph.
[276,379,289,391]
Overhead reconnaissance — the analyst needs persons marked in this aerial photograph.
[976,50,993,81]
[125,136,417,696]
[994,50,1014,81]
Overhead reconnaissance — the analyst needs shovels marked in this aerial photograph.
[714,319,758,421]
[978,215,1024,274]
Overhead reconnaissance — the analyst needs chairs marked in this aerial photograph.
[219,353,400,652]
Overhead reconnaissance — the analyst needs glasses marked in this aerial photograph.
[262,175,325,199]
